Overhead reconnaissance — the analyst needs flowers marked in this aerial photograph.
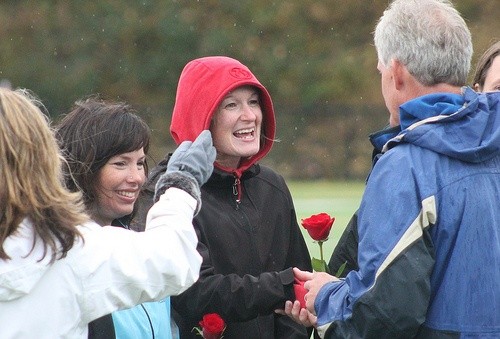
[300,212,349,276]
[189,314,230,339]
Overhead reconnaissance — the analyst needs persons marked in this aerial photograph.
[129,55,314,339]
[53,92,188,338]
[273,0,500,339]
[0,87,217,339]
